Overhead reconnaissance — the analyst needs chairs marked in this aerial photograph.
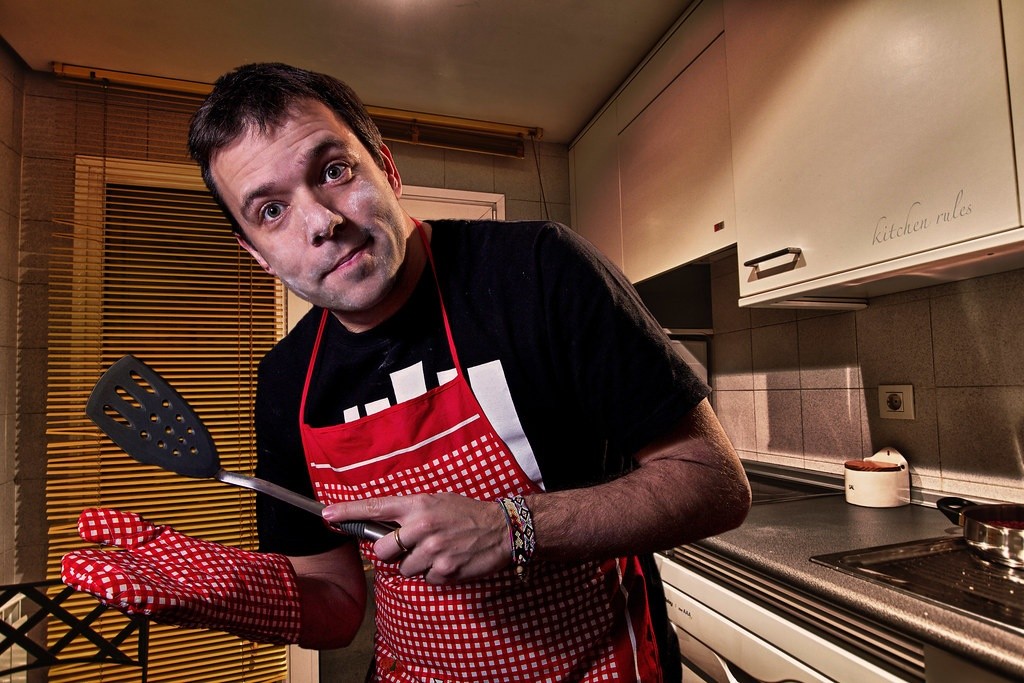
[0,577,149,682]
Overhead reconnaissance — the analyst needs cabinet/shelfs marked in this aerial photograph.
[617,30,726,285]
[723,0,1024,307]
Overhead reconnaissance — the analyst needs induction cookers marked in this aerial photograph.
[811,535,1024,634]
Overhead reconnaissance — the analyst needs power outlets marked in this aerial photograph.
[878,384,915,419]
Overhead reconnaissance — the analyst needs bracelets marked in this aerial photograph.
[496,495,535,584]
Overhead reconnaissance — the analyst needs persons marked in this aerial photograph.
[61,62,752,683]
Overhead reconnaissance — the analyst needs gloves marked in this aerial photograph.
[61,506,301,648]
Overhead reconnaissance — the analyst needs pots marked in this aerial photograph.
[937,495,1024,569]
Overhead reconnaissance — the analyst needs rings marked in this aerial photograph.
[394,528,407,552]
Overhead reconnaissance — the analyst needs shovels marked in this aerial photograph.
[83,354,400,541]
